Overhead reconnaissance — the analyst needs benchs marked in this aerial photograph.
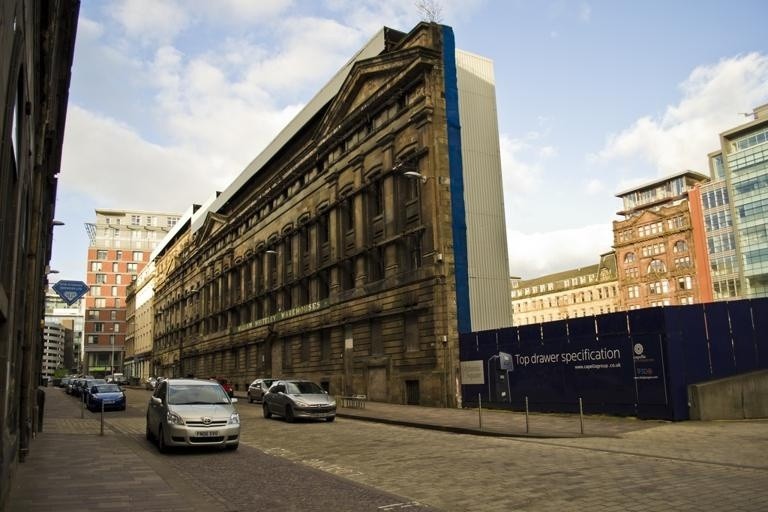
[334,394,366,408]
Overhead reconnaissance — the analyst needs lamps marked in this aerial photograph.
[44,265,60,276]
[266,250,281,255]
[404,172,427,183]
[43,221,65,228]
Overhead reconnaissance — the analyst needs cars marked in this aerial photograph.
[248,378,274,405]
[59,371,167,400]
[211,375,233,397]
[144,376,240,454]
[262,380,336,425]
[87,384,126,411]
